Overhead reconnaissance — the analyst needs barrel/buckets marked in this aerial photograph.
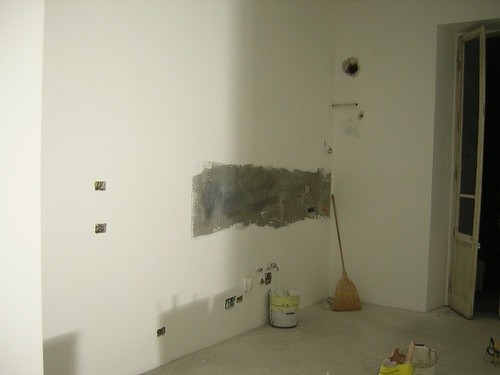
[268,289,300,328]
